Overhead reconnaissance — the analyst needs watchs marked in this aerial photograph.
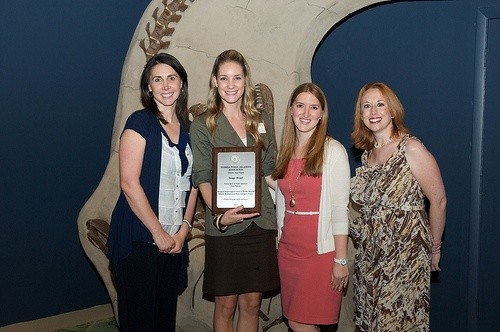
[334,258,348,265]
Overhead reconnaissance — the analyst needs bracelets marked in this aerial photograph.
[182,218,192,233]
[211,214,229,233]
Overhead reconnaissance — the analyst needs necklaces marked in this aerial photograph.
[373,139,394,149]
[286,161,306,208]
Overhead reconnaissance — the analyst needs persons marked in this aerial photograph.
[274,83,349,332]
[350,82,446,332]
[189,48,278,332]
[109,53,199,332]
